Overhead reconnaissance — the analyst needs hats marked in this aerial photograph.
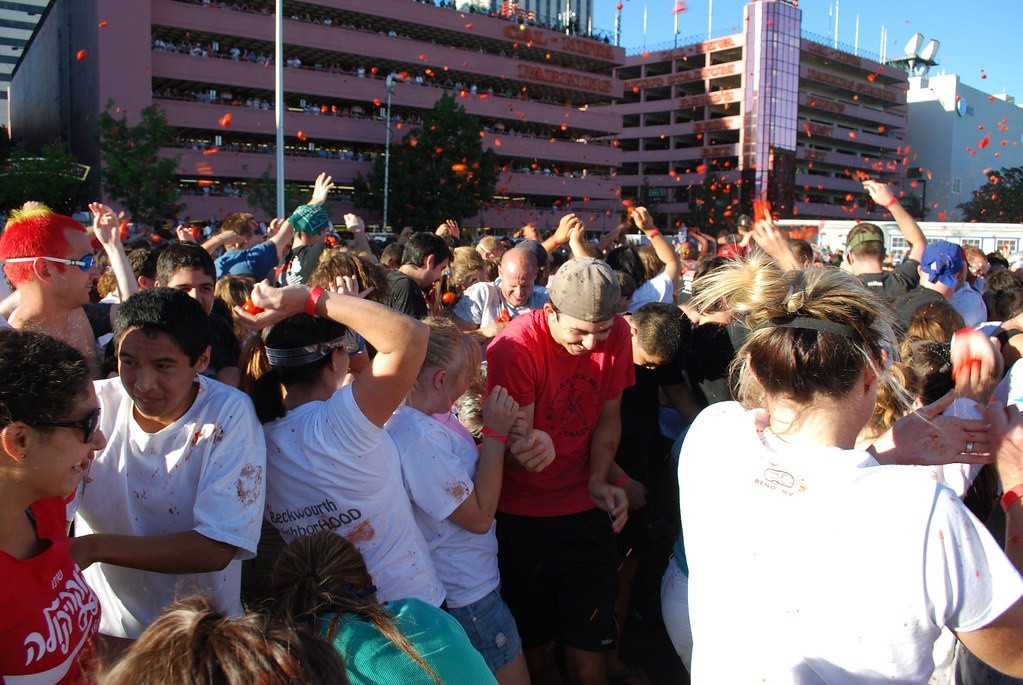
[547,256,620,321]
[735,215,752,227]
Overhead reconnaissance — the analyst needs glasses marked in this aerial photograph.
[16,407,102,443]
[873,338,894,371]
[841,251,852,267]
[78,256,97,271]
[330,332,364,356]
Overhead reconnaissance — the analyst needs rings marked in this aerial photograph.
[986,424,992,430]
[965,441,974,454]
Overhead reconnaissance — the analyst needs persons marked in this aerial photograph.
[0,172,1023,685]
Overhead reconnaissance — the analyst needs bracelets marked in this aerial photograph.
[480,425,512,444]
[1000,485,1023,512]
[614,474,630,486]
[883,196,899,210]
[549,232,560,250]
[305,284,323,318]
[64,487,79,504]
[349,225,365,237]
[646,228,660,242]
